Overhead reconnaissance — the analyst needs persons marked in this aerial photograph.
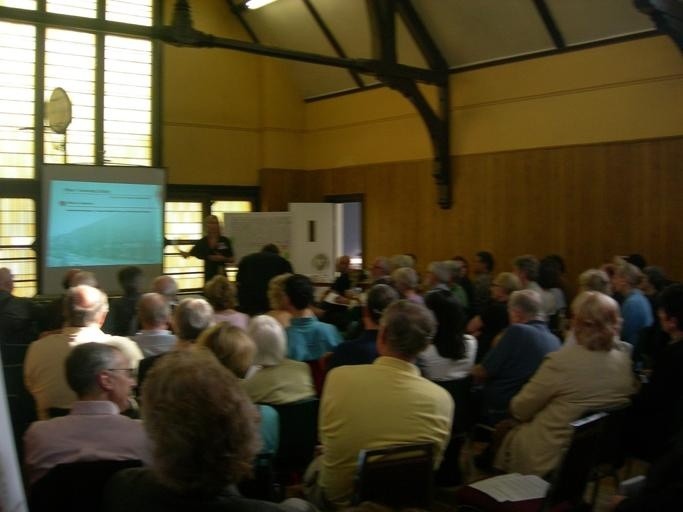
[178,215,234,285]
[0,244,423,473]
[419,242,683,476]
[302,300,453,511]
[88,349,314,512]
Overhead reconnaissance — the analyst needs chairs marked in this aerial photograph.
[32,460,143,511]
[558,401,633,509]
[255,400,439,510]
[429,376,475,460]
